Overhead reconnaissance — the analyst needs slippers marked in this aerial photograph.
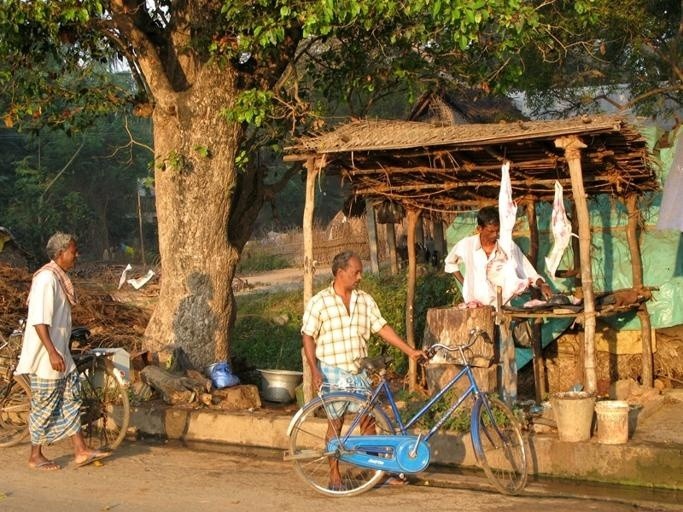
[72,448,112,468]
[374,476,409,487]
[32,459,61,471]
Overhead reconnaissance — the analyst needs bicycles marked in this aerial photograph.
[284,326,528,497]
[0,314,131,459]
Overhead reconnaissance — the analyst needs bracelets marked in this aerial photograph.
[541,280,550,287]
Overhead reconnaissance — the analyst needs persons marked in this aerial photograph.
[300,250,428,490]
[445,206,554,404]
[18,233,112,472]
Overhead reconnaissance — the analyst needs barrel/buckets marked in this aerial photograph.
[549,390,597,444]
[593,400,631,445]
[255,368,304,403]
